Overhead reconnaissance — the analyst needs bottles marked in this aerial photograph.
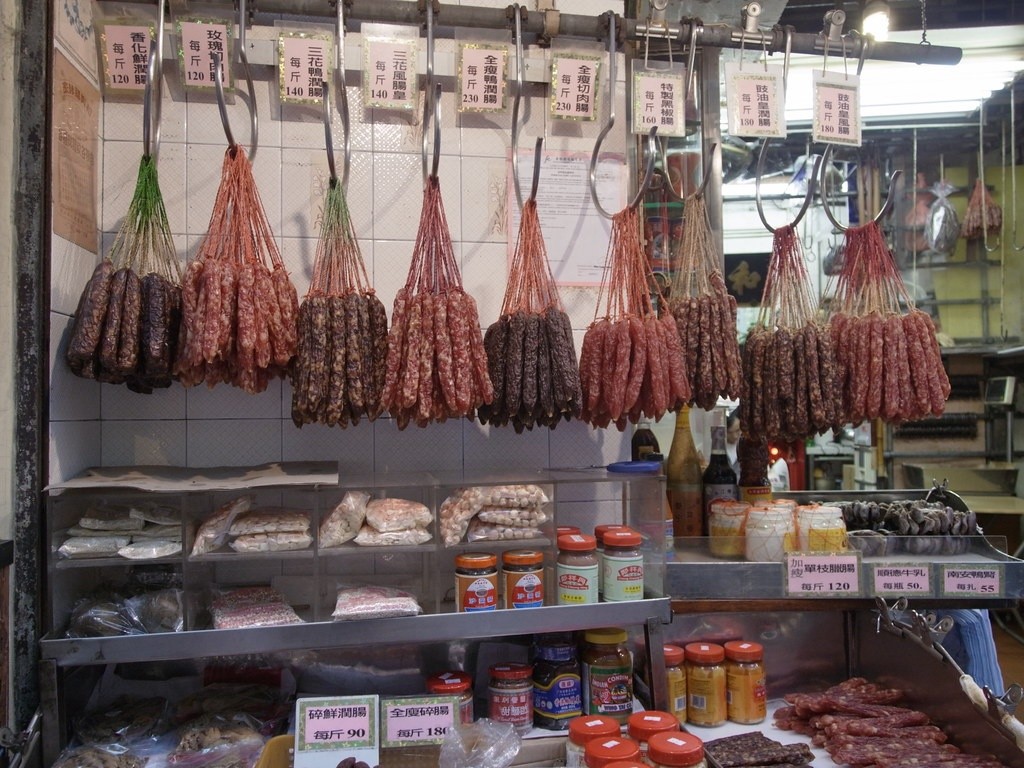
[742,493,798,562]
[425,629,708,768]
[662,640,767,727]
[453,525,644,613]
[736,433,773,506]
[798,505,849,551]
[701,406,737,545]
[666,402,704,547]
[705,499,751,557]
[640,475,674,561]
[630,422,663,529]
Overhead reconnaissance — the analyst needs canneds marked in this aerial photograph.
[684,642,727,728]
[532,639,583,730]
[454,551,500,613]
[583,627,634,725]
[565,711,708,768]
[724,640,767,724]
[707,499,847,561]
[542,523,644,604]
[425,671,474,726]
[500,548,544,611]
[642,645,687,728]
[486,661,534,736]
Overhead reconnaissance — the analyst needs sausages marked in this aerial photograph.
[63,258,952,443]
[701,730,817,768]
[771,678,1010,768]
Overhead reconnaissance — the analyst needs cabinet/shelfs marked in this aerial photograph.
[44,469,668,637]
[901,182,1004,341]
